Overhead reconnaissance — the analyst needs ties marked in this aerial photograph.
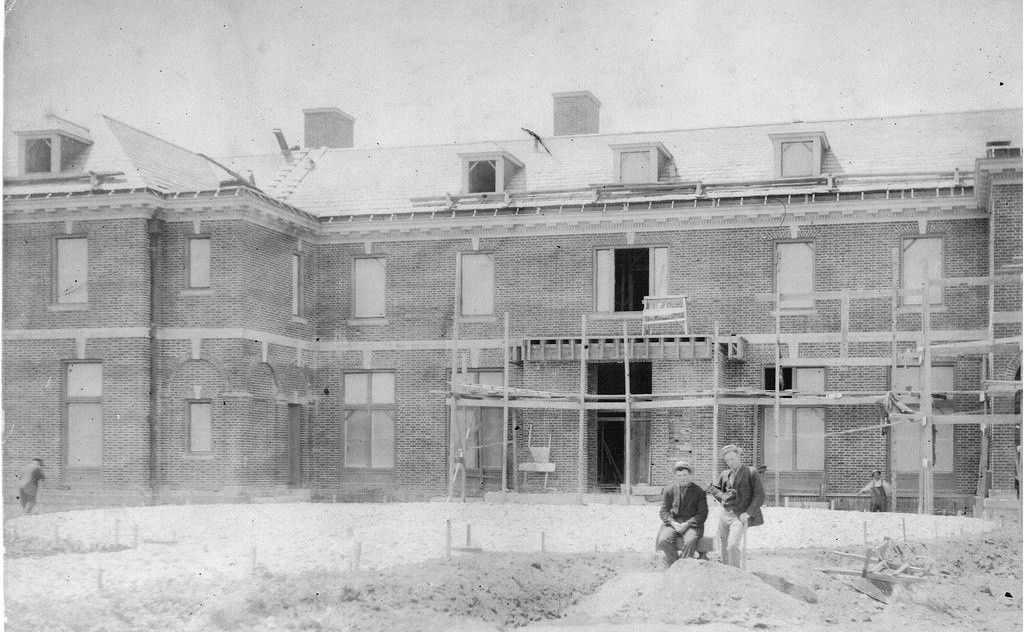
[730,469,735,486]
[680,487,684,501]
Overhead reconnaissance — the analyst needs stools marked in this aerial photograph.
[655,537,713,560]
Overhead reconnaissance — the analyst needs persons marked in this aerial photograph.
[656,461,708,567]
[708,444,764,568]
[858,469,892,512]
[18,458,46,513]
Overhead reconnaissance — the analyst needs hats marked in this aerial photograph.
[32,455,41,462]
[719,444,742,456]
[871,468,880,476]
[673,461,694,476]
